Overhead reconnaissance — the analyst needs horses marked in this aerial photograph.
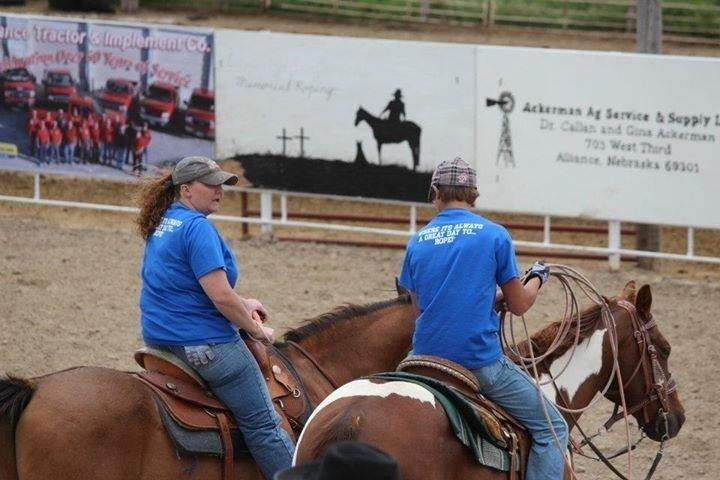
[290,279,687,480]
[0,275,418,480]
[353,105,421,174]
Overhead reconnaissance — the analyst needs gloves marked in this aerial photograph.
[521,258,552,290]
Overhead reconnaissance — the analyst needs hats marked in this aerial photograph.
[425,155,479,202]
[391,88,405,98]
[171,155,239,186]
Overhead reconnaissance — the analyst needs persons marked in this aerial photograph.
[27,106,151,176]
[395,162,571,479]
[380,88,406,122]
[131,153,296,480]
[272,440,402,479]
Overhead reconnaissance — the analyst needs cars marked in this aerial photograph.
[0,66,215,142]
